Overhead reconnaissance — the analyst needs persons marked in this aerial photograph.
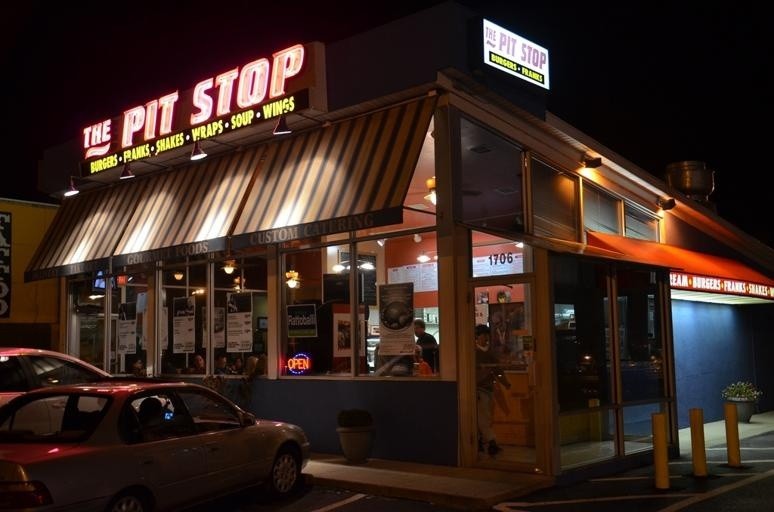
[474,322,511,456]
[113,345,268,374]
[373,319,438,376]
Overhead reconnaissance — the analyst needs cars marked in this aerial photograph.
[0,378,310,512]
[551,325,668,400]
[0,347,173,439]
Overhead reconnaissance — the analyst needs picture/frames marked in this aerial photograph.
[336,248,380,311]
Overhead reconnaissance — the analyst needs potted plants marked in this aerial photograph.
[334,406,375,462]
[722,381,763,422]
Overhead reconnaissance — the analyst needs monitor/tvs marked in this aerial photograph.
[91,272,118,294]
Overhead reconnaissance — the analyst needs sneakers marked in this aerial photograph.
[487,444,502,456]
[479,439,485,452]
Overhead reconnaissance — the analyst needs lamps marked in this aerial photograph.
[272,111,330,137]
[376,233,422,247]
[62,175,113,196]
[190,137,243,162]
[119,158,173,182]
[282,242,299,290]
[580,154,603,169]
[656,196,675,210]
[422,176,437,206]
[220,238,237,275]
